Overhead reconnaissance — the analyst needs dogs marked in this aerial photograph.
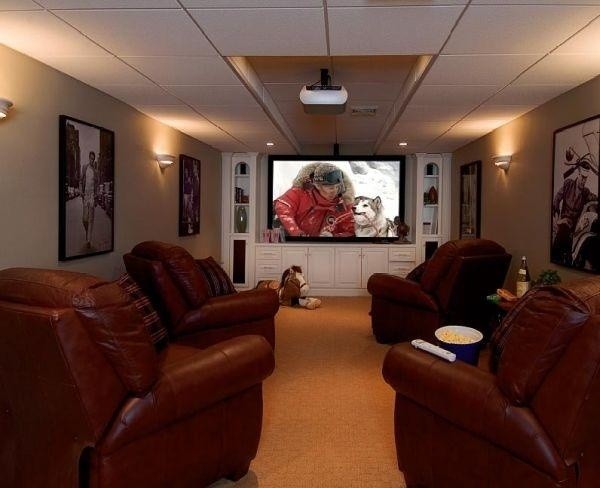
[351,196,398,237]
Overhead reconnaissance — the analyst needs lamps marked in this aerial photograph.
[156,154,176,170]
[491,155,512,170]
[0,97,13,121]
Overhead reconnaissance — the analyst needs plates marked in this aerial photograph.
[435,325,483,347]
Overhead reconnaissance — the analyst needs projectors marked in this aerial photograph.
[300,85,348,106]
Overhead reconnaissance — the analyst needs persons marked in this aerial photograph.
[183,164,199,234]
[273,163,355,237]
[79,151,100,249]
[552,162,597,267]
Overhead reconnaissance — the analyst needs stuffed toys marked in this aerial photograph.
[257,265,321,309]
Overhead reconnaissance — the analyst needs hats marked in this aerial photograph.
[314,163,343,184]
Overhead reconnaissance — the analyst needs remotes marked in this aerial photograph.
[411,339,456,362]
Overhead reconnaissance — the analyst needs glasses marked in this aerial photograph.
[313,170,344,183]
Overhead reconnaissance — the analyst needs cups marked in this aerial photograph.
[259,228,270,243]
[238,189,243,203]
[240,163,247,174]
[271,227,280,243]
[427,164,433,175]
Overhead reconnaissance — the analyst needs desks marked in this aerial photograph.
[486,289,520,340]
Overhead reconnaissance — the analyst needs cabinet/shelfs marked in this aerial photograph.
[413,152,442,271]
[228,152,259,287]
[388,244,416,279]
[255,245,282,286]
[281,244,335,289]
[335,245,389,289]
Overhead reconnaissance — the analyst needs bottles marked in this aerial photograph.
[516,255,531,297]
[279,224,285,243]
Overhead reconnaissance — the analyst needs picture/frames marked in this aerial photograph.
[459,160,481,239]
[550,114,600,275]
[58,115,115,262]
[179,153,201,237]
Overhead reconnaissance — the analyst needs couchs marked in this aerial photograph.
[121,239,280,354]
[366,238,513,345]
[0,266,276,488]
[381,276,600,487]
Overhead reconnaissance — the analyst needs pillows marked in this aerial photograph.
[403,259,429,283]
[196,255,238,297]
[489,283,545,375]
[113,271,169,345]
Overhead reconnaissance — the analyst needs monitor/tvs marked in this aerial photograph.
[267,153,406,242]
